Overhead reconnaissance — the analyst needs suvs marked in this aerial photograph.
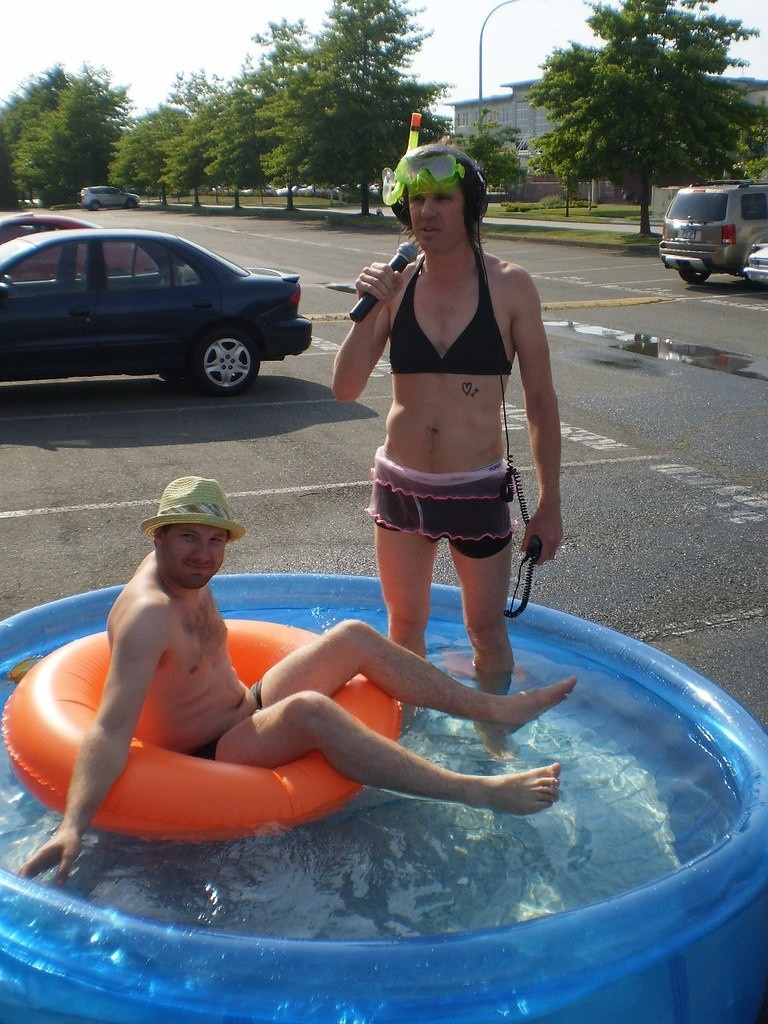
[79,186,141,212]
[657,178,768,283]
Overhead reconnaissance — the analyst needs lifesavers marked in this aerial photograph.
[2,618,403,841]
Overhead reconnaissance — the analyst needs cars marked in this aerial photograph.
[742,243,768,286]
[242,182,383,200]
[0,211,158,284]
[0,228,315,399]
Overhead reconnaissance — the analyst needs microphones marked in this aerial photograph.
[349,242,418,323]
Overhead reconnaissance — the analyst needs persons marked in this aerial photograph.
[333,146,563,672]
[19,476,577,886]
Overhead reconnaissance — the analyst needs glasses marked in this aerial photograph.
[382,154,466,206]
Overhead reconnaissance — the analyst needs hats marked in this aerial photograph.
[391,145,488,227]
[139,477,246,543]
